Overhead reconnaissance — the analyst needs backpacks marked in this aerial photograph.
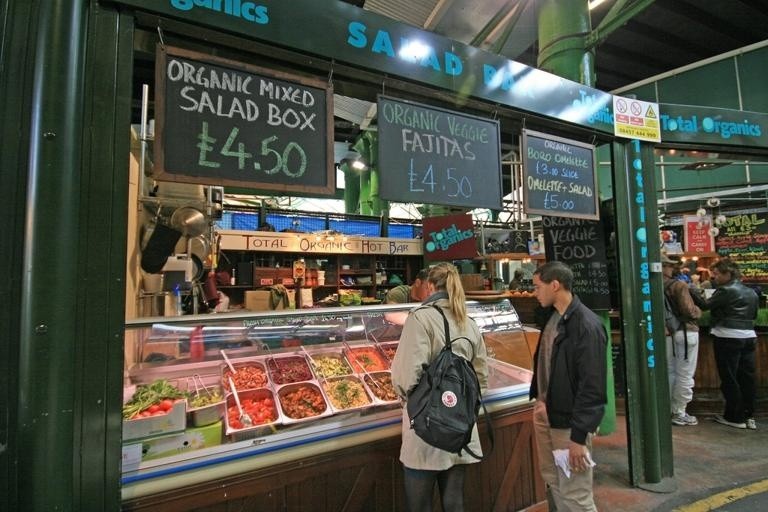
[664,279,689,337]
[406,305,496,460]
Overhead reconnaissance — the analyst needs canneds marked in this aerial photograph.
[305,268,319,287]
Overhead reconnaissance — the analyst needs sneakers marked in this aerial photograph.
[672,413,699,426]
[747,418,758,431]
[713,413,747,429]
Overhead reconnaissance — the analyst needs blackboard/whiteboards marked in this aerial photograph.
[542,216,612,312]
[377,93,504,211]
[153,42,336,195]
[712,207,768,284]
[611,343,627,400]
[521,128,600,222]
[683,215,715,252]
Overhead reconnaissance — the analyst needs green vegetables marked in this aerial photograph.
[123,379,188,419]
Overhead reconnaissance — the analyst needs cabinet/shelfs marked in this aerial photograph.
[215,250,412,312]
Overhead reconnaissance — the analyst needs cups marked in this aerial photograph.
[316,270,326,286]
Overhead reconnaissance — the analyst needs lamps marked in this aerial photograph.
[677,155,734,236]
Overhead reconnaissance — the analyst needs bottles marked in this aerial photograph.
[229,268,236,286]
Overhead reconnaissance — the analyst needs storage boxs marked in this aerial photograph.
[243,288,297,311]
[121,400,222,469]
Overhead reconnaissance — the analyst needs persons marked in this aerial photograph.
[363,267,435,342]
[659,254,704,426]
[688,260,760,430]
[528,261,608,512]
[509,268,528,291]
[390,262,488,511]
[670,255,717,291]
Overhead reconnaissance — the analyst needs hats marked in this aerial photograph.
[660,253,678,264]
